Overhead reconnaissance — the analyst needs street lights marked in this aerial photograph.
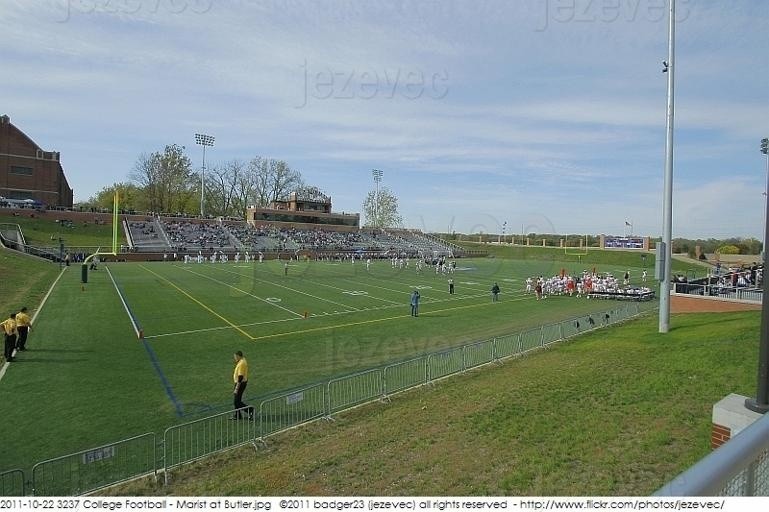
[372,169,383,229]
[195,133,215,216]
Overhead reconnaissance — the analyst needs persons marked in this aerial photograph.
[526,269,648,301]
[0,314,17,362]
[411,290,420,317]
[749,262,757,285]
[15,307,33,352]
[672,274,688,292]
[0,195,456,276]
[448,277,455,295]
[228,351,253,421]
[489,281,500,302]
